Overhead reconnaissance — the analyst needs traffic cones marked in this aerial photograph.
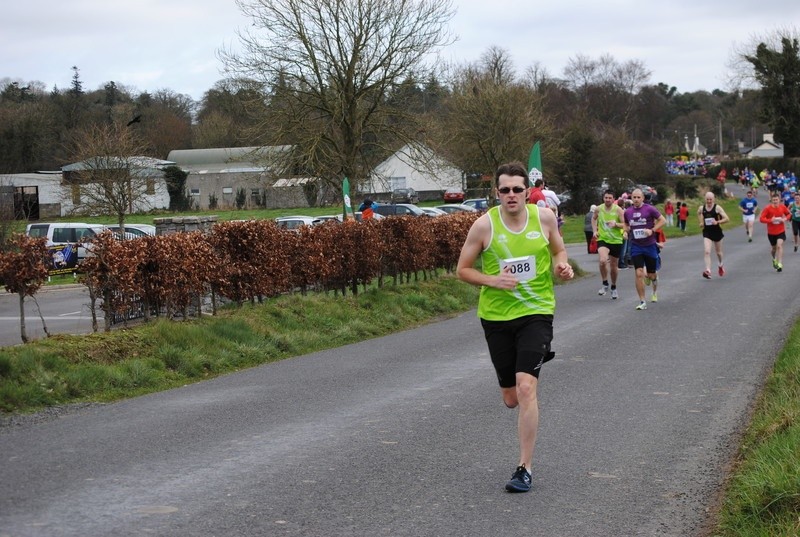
[730,192,736,201]
[723,188,728,197]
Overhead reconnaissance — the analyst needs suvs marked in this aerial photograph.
[601,176,658,196]
[274,214,323,235]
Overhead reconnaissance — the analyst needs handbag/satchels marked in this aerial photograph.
[589,236,597,253]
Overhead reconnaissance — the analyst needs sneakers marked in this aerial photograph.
[598,285,608,296]
[505,463,532,492]
[777,263,782,272]
[772,257,778,269]
[703,271,711,279]
[718,265,725,277]
[542,350,555,364]
[636,300,647,310]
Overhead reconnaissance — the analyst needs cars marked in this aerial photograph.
[554,187,607,203]
[390,189,413,203]
[444,188,466,203]
[418,204,478,218]
[315,211,385,225]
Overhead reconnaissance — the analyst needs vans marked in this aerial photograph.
[24,222,157,260]
[359,203,425,218]
[462,198,501,212]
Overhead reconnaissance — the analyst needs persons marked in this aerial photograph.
[360,198,374,222]
[584,157,800,311]
[455,159,574,492]
[528,179,546,207]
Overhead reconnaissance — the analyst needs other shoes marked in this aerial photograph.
[643,276,651,286]
[652,294,657,302]
[748,238,753,242]
[793,245,798,252]
[610,288,618,299]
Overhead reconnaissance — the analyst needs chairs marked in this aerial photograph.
[83,230,89,237]
[76,231,81,240]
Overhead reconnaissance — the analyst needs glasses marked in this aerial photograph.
[498,186,525,194]
